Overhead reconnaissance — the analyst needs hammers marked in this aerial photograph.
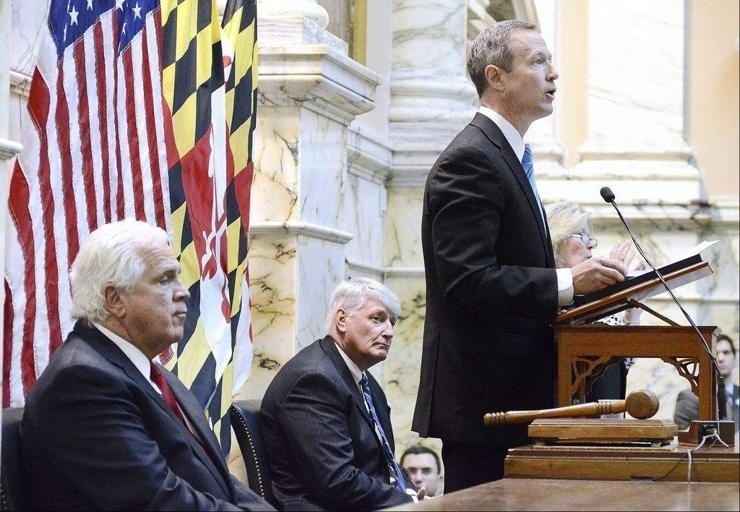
[483,389,661,426]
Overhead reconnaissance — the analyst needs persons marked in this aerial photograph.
[543,200,649,403]
[400,447,441,501]
[16,220,279,512]
[674,334,740,433]
[260,278,426,512]
[412,18,630,495]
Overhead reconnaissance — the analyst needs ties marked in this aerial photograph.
[359,374,407,492]
[150,362,185,426]
[523,144,547,240]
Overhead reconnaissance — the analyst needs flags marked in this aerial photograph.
[1,1,257,465]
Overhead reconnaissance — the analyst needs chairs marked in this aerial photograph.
[229,398,284,512]
[2,405,40,512]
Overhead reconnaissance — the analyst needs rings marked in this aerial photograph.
[620,256,624,262]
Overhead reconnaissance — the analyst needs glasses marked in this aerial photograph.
[571,232,598,246]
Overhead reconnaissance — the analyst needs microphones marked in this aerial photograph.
[600,187,728,420]
